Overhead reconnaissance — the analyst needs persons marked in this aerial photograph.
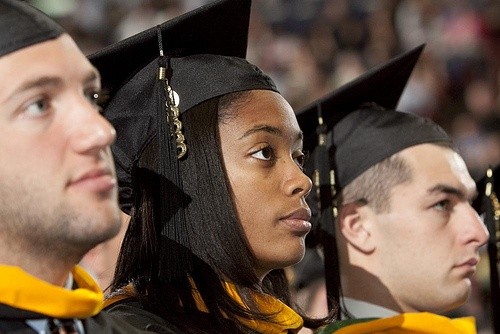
[294,41,489,334]
[0,0,121,334]
[85,0,313,334]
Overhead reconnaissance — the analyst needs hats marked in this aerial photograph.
[84,0,282,282]
[294,43,450,323]
[0,0,65,58]
